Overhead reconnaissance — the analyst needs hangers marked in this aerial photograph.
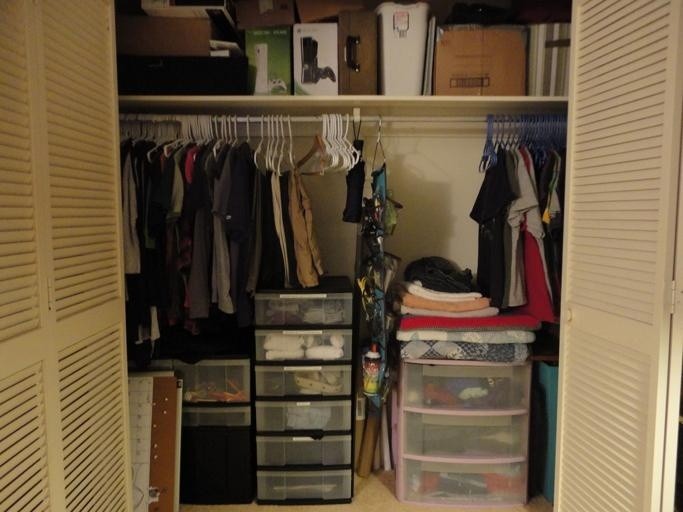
[478,114,567,172]
[121,114,387,177]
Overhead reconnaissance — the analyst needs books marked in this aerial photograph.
[420,13,439,97]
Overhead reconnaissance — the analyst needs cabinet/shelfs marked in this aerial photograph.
[0,0,683,512]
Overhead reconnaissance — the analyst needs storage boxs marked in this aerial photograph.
[389,358,556,507]
[375,1,429,95]
[115,0,364,94]
[434,27,527,97]
[130,277,357,506]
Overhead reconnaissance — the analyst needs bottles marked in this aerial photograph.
[362,344,381,396]
